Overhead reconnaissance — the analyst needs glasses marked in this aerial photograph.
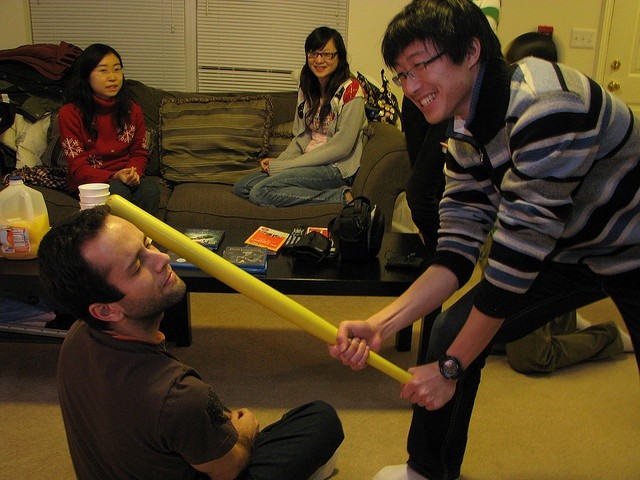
[94,66,128,73]
[392,52,445,86]
[306,51,338,60]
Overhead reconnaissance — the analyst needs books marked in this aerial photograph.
[306,227,336,255]
[245,225,289,255]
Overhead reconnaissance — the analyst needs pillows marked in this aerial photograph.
[269,119,294,159]
[158,93,276,185]
[46,106,69,167]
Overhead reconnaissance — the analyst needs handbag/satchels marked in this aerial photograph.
[292,230,331,276]
[328,195,384,272]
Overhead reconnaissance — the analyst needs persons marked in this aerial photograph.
[233,27,368,208]
[38,205,345,479]
[326,1,640,478]
[506,32,636,374]
[58,44,160,217]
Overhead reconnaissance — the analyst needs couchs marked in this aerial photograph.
[0,90,414,234]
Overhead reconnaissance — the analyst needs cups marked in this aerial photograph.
[78,183,110,195]
[79,202,106,210]
[79,192,111,203]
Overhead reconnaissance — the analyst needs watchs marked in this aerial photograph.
[439,353,467,380]
